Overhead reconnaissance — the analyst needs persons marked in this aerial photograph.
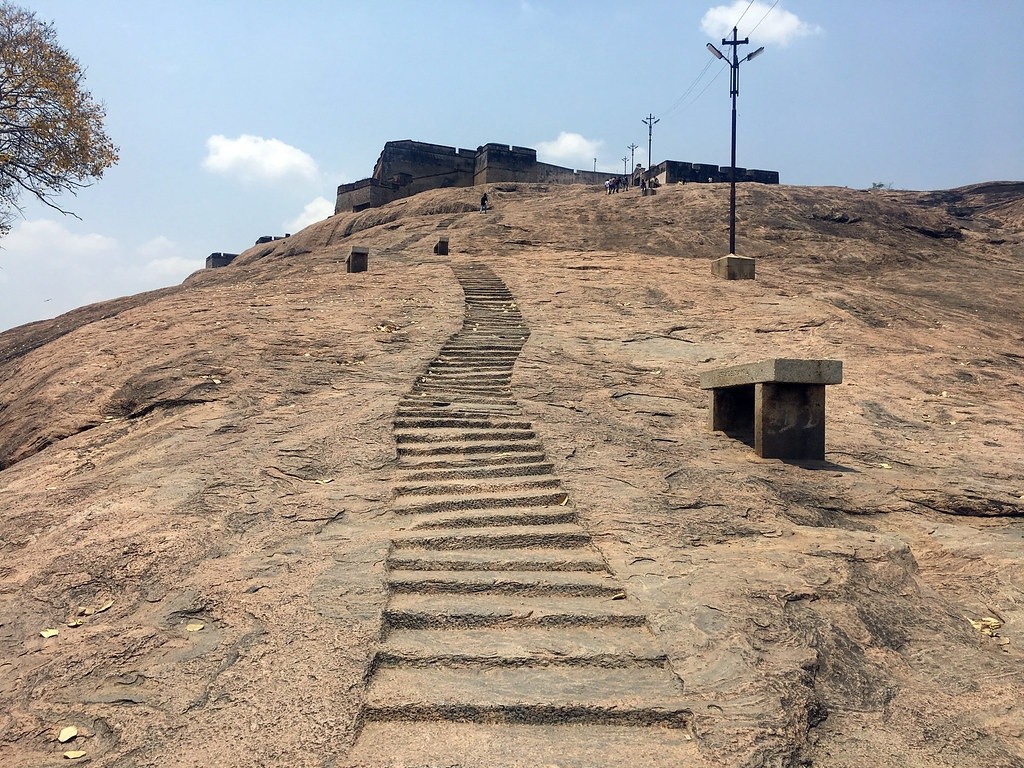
[480,192,488,214]
[604,176,629,195]
[708,176,713,183]
[653,175,662,188]
[640,179,648,196]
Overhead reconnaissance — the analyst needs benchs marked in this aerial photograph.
[700,359,842,462]
[345,246,369,273]
[433,238,449,255]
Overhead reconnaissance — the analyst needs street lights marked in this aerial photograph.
[642,115,660,190]
[622,158,630,180]
[627,145,638,185]
[706,26,766,252]
